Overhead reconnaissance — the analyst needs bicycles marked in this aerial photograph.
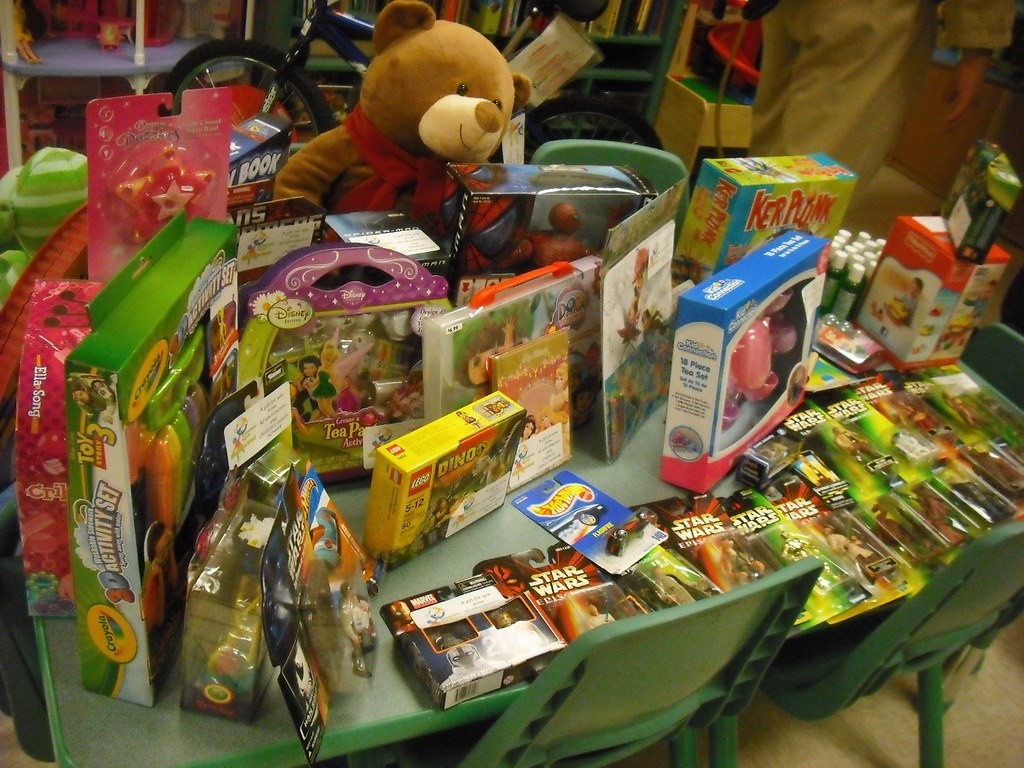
[153,0,665,164]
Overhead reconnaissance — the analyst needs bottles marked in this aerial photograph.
[819,229,886,320]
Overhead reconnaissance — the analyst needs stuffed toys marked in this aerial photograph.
[273,0,534,237]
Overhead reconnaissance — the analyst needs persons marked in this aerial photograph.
[963,279,997,328]
[339,582,378,676]
[750,0,1016,230]
[494,390,1024,630]
[894,278,923,326]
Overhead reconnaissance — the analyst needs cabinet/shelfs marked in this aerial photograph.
[0,0,688,188]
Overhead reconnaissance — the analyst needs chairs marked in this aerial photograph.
[771,524,1024,768]
[348,556,825,768]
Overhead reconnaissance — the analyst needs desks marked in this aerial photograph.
[15,315,1024,768]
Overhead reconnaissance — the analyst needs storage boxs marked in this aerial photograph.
[942,137,1024,258]
[362,391,526,568]
[669,153,857,286]
[59,211,242,704]
[854,214,1010,374]
[227,113,293,204]
[663,230,831,491]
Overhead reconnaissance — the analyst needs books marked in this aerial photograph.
[464,0,673,39]
